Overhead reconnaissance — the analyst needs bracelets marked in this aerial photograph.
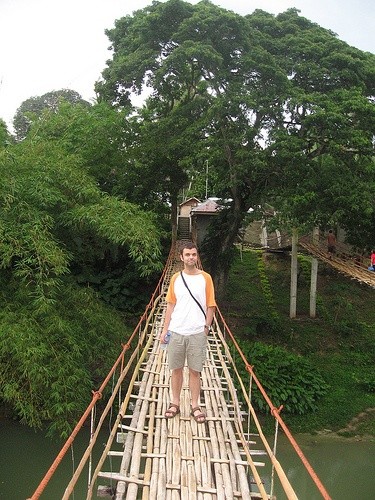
[205,325,210,331]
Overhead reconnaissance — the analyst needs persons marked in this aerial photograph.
[370,249,375,272]
[326,230,337,261]
[159,243,217,423]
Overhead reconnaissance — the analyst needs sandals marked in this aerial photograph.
[165,402,180,418]
[190,405,205,423]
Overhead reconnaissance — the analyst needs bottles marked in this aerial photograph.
[160,333,172,351]
